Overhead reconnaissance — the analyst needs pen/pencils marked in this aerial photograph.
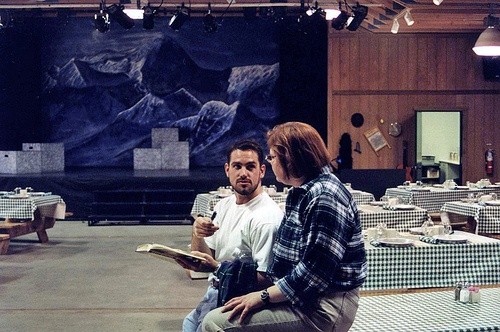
[210,212,217,224]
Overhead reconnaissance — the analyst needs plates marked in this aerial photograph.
[460,199,477,202]
[218,194,228,197]
[371,202,384,205]
[485,200,500,205]
[378,238,415,247]
[408,228,424,235]
[412,188,430,191]
[209,191,220,194]
[433,235,469,243]
[457,186,470,189]
[395,205,416,209]
[433,185,444,187]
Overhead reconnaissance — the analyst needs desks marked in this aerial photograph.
[345,185,500,235]
[348,287,500,332]
[362,227,500,290]
[0,191,66,244]
[191,192,288,220]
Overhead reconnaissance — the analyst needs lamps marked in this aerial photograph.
[471,0,500,57]
[94,0,368,34]
[432,0,443,5]
[403,9,414,27]
[391,18,399,34]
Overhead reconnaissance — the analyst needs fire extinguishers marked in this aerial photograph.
[486,149,495,175]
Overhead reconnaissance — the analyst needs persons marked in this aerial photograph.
[199,121,368,332]
[181,140,286,332]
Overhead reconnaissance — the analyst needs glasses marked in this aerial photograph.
[265,154,277,161]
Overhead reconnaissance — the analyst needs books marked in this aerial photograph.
[135,242,208,264]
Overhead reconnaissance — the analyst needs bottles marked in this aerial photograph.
[469,286,480,303]
[459,287,469,303]
[455,286,461,300]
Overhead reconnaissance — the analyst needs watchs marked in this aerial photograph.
[260,288,272,306]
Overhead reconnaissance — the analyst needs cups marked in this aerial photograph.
[363,229,378,239]
[417,181,421,187]
[345,186,351,191]
[428,227,439,237]
[406,181,410,186]
[435,226,445,235]
[386,229,398,238]
[389,199,397,207]
[440,222,451,235]
[375,223,388,238]
[267,188,276,196]
[467,192,497,202]
